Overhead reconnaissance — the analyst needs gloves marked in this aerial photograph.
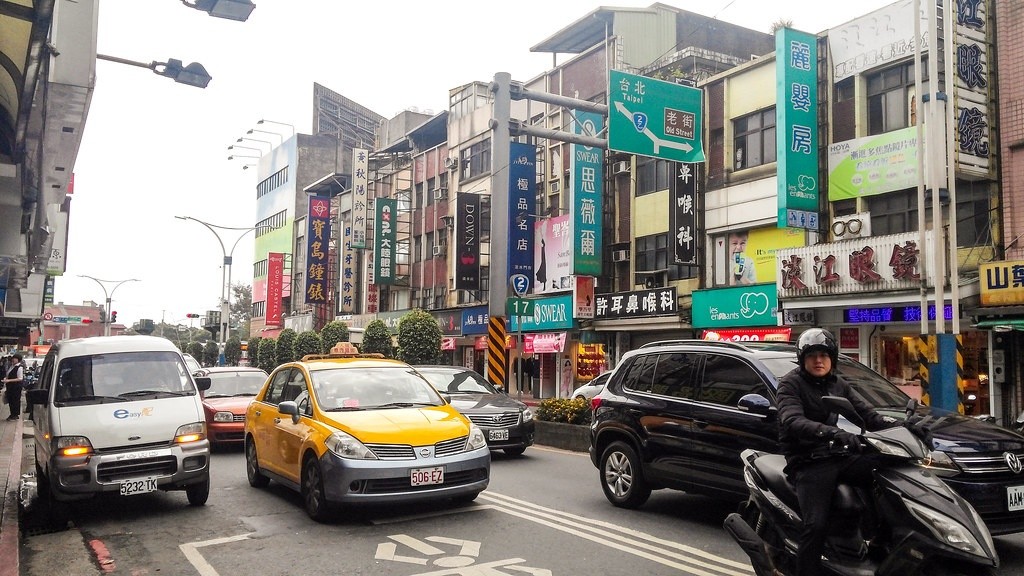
[910,422,935,453]
[833,430,867,451]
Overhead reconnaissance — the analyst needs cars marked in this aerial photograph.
[193,366,270,447]
[243,342,503,524]
[411,365,534,454]
[571,370,616,402]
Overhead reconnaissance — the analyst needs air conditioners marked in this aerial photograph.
[433,187,449,199]
[612,250,630,262]
[445,157,458,169]
[432,245,444,256]
[444,218,453,227]
[612,160,631,174]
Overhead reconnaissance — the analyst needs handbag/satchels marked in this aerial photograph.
[1,387,9,404]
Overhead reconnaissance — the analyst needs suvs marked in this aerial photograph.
[590,338,1024,544]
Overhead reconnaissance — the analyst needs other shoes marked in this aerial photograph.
[869,542,890,558]
[6,415,19,421]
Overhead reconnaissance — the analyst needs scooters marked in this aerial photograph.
[725,393,1001,576]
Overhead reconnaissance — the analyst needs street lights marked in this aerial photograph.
[173,215,281,366]
[79,273,144,337]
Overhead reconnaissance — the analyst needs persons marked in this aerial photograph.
[776,326,936,576]
[728,230,758,285]
[513,352,535,395]
[1,354,24,423]
[561,360,573,397]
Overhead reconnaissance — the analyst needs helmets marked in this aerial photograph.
[797,327,838,368]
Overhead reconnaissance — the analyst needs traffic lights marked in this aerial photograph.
[83,320,94,323]
[187,313,200,318]
[110,312,117,323]
[99,310,106,323]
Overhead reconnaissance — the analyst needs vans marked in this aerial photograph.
[27,336,210,510]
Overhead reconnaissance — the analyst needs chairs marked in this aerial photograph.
[284,382,302,400]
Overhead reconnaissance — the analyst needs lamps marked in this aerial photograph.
[236,136,272,151]
[246,128,282,144]
[257,118,294,136]
[179,0,257,22]
[243,164,256,171]
[95,53,213,89]
[227,154,260,160]
[227,145,262,157]
